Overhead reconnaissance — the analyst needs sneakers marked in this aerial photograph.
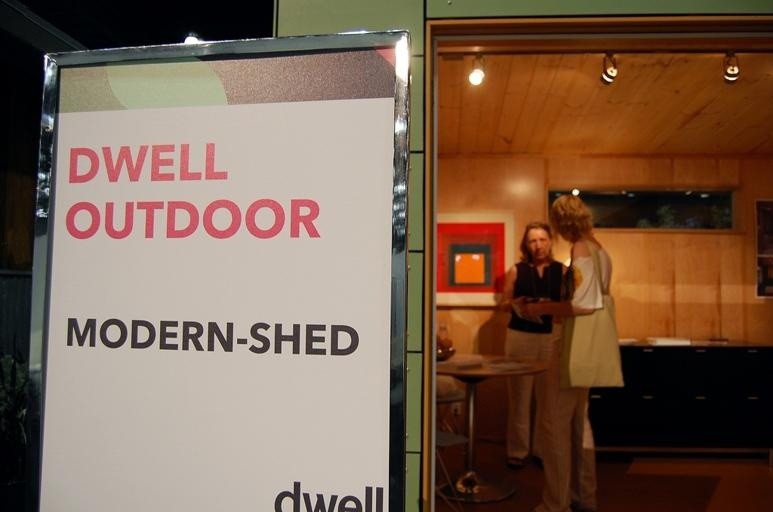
[570,499,594,512]
[506,454,544,471]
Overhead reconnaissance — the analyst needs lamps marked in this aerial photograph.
[468,49,742,89]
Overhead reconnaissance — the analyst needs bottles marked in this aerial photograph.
[435,321,454,360]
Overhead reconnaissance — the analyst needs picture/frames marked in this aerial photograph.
[434,211,516,310]
[15,27,415,512]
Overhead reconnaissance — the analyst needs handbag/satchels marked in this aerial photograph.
[560,239,625,391]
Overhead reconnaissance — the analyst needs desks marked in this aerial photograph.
[436,351,549,505]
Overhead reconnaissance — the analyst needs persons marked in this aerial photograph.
[501,220,570,470]
[515,194,629,511]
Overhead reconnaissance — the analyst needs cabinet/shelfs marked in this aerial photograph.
[587,346,773,446]
